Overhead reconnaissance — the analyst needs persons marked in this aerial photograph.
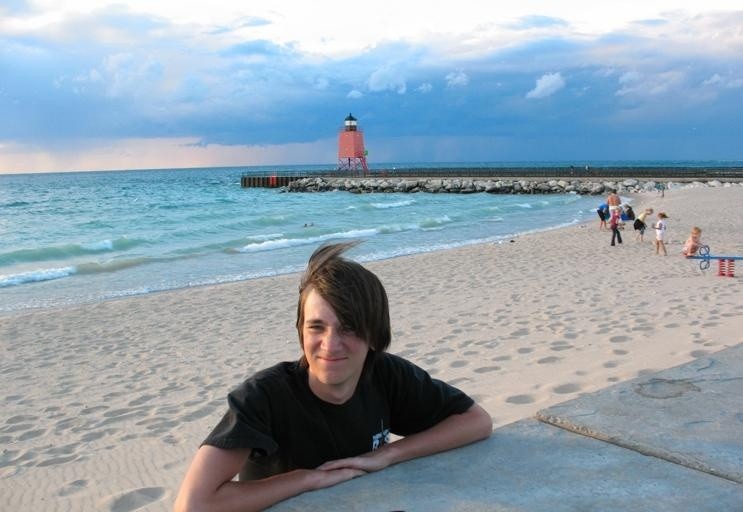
[164,242,491,512]
[651,212,669,256]
[681,226,705,257]
[596,186,653,246]
